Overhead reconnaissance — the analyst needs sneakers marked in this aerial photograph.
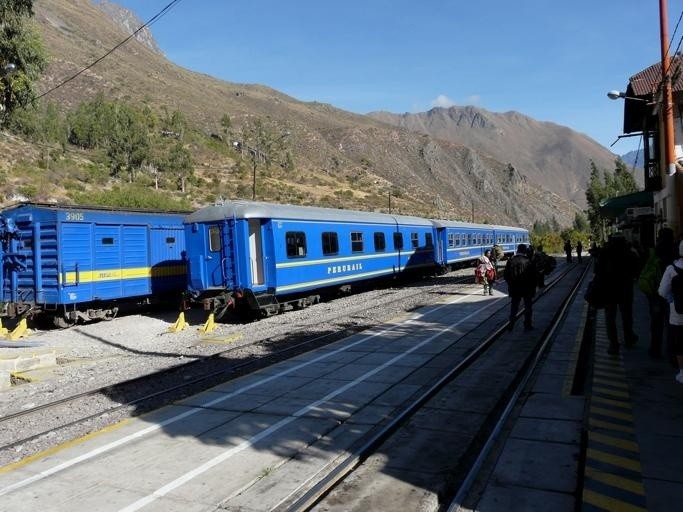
[608,334,640,354]
[648,344,683,385]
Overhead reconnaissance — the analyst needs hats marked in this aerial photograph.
[517,245,530,254]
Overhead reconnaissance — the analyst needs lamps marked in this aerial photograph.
[607,90,649,103]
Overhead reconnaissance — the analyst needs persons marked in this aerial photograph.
[525,245,533,260]
[646,227,674,358]
[575,240,582,262]
[532,245,548,297]
[591,233,646,289]
[593,231,640,356]
[503,243,539,332]
[491,244,504,262]
[563,239,573,264]
[479,250,496,296]
[654,238,682,388]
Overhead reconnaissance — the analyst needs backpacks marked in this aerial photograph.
[639,247,666,295]
[671,261,683,315]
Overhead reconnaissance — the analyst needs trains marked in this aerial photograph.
[168,199,531,334]
[0,200,197,341]
